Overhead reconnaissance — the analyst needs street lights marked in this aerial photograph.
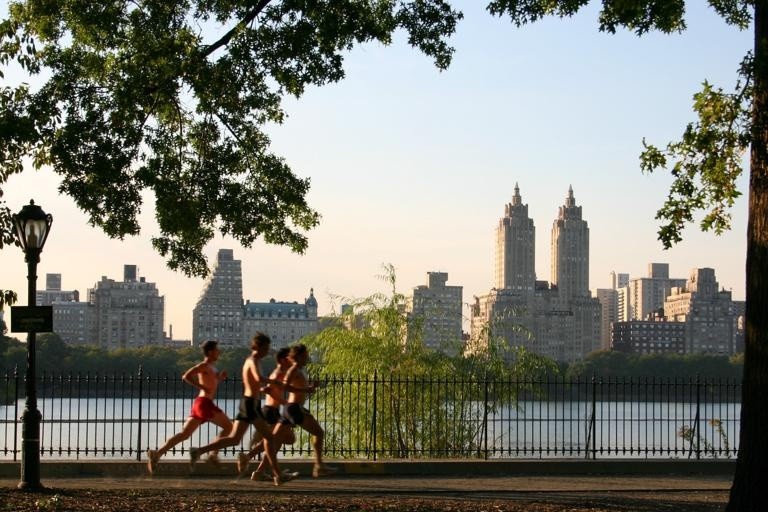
[11,198,53,492]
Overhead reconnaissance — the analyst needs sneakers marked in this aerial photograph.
[190,447,300,487]
[147,450,159,474]
[312,466,339,478]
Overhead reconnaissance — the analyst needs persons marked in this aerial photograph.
[238,346,298,481]
[250,344,341,481]
[147,340,233,473]
[189,332,316,486]
